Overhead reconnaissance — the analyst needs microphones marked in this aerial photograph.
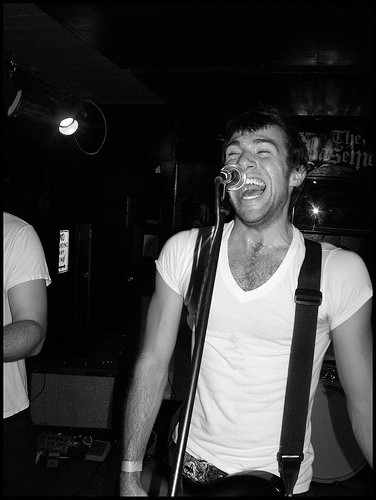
[215,165,246,190]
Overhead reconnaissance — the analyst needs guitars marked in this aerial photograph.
[139,462,293,500]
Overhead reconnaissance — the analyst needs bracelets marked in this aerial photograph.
[121,460,142,472]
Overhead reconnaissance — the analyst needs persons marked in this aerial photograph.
[119,104,376,497]
[0,210,52,499]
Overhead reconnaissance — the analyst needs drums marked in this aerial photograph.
[312,365,367,484]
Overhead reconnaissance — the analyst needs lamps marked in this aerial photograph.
[53,101,87,143]
